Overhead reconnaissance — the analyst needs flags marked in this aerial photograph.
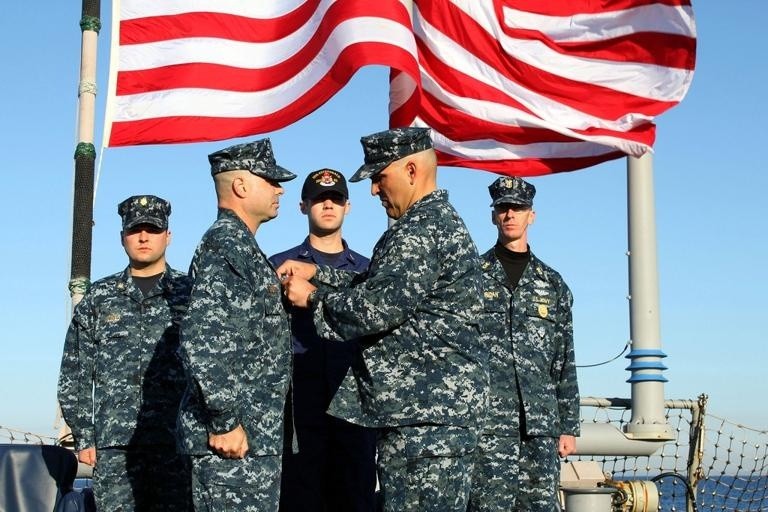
[102,0,698,178]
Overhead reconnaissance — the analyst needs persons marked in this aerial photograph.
[469,173,581,511]
[174,137,298,512]
[277,126,488,511]
[267,167,378,510]
[56,194,192,512]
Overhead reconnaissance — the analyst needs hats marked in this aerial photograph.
[303,169,349,199]
[117,195,173,233]
[207,138,297,182]
[349,126,432,184]
[488,176,536,208]
